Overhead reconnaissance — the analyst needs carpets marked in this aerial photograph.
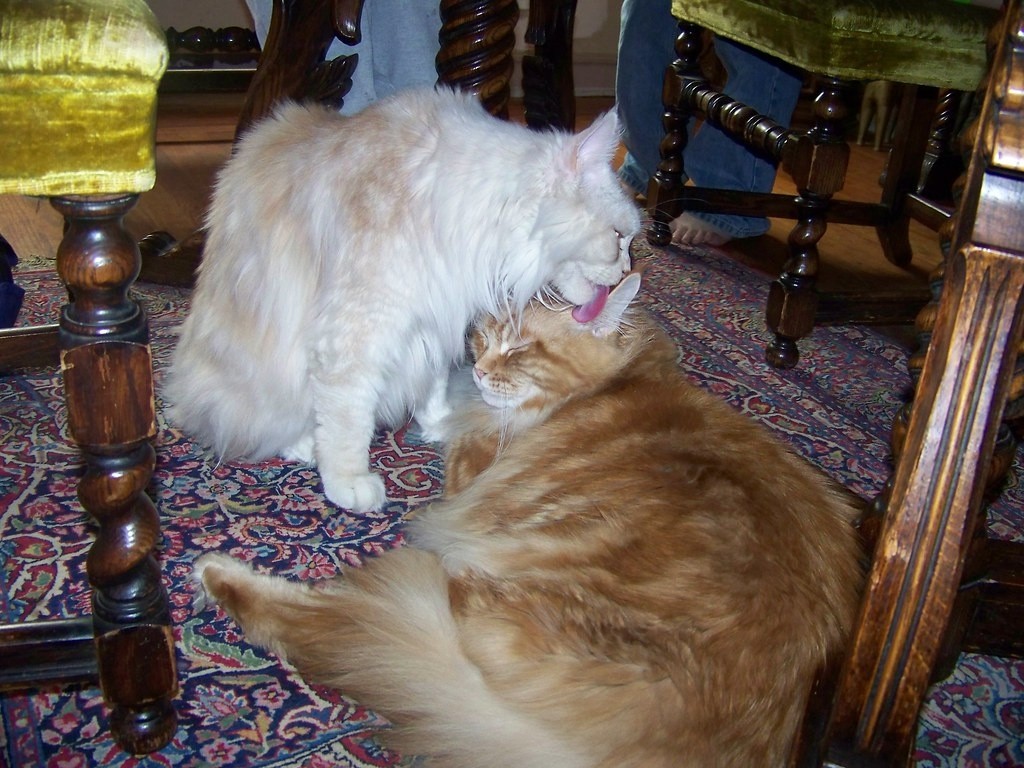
[1,225,1023,767]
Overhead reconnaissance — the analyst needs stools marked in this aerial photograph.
[0,0,185,750]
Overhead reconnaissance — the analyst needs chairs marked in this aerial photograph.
[647,0,1005,370]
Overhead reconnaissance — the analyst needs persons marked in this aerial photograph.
[857,79,891,152]
[245,0,442,116]
[613,0,803,248]
